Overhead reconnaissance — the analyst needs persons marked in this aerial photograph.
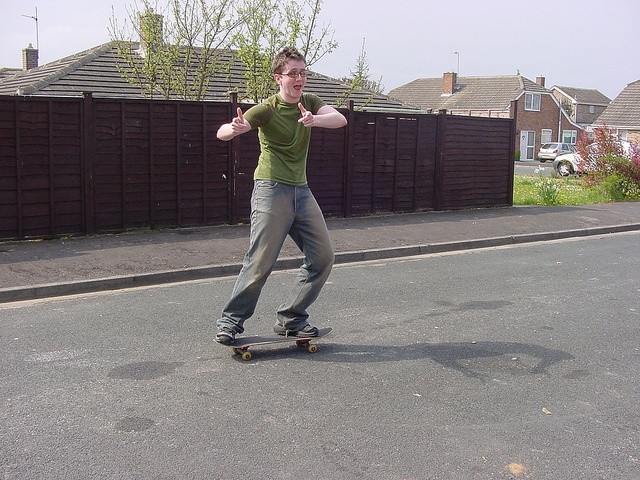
[216,46,348,346]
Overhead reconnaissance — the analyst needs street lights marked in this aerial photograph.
[454,51,459,77]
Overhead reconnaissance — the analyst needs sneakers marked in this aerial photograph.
[273,320,319,338]
[216,326,235,345]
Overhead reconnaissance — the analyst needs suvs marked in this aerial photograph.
[538,142,577,163]
[552,141,637,177]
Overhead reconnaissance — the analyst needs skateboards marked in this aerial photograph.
[213,327,333,361]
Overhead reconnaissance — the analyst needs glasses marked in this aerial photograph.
[276,71,306,78]
